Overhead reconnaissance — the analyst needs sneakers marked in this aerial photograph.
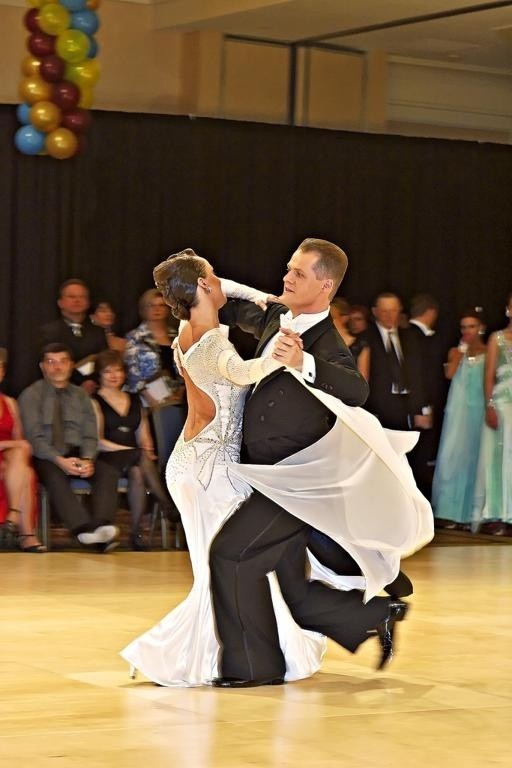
[78,523,121,553]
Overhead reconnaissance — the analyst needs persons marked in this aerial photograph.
[206,233,415,690]
[142,246,329,688]
[0,268,512,555]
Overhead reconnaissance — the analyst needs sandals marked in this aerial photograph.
[1,508,21,538]
[17,535,49,554]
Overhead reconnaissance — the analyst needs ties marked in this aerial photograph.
[51,388,70,456]
[387,332,408,393]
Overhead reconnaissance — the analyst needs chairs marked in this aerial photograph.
[150,402,187,553]
[29,476,146,554]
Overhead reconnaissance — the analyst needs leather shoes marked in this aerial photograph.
[212,669,286,688]
[376,603,405,671]
[130,527,148,548]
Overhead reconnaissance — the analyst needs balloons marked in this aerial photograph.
[15,0,103,161]
